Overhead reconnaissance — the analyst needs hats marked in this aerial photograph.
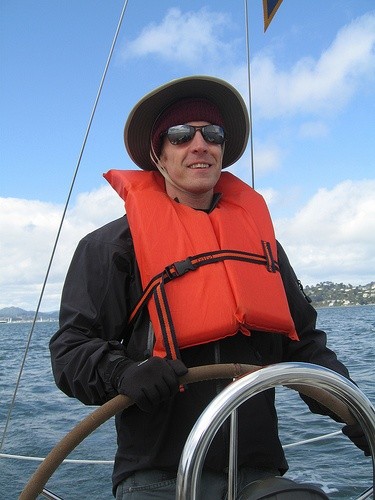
[124,75,250,170]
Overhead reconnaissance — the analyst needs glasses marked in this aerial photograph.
[160,124,225,145]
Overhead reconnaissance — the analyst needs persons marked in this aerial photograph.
[47,73,375,500]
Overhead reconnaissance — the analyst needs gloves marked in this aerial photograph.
[110,356,186,412]
[342,422,372,457]
